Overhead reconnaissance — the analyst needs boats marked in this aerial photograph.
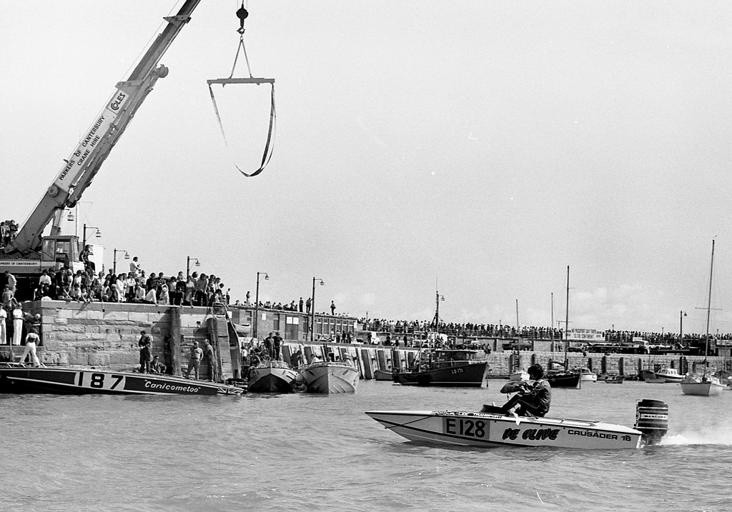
[364,395,668,452]
[2,364,248,399]
[543,367,732,395]
[247,359,299,393]
[375,347,491,388]
[296,362,360,395]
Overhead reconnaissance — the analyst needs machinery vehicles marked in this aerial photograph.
[0,0,278,296]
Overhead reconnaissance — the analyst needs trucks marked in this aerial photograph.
[412,330,450,347]
[354,330,388,344]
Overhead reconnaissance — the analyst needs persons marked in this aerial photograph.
[496,361,553,416]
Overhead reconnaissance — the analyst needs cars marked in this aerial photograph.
[502,338,533,352]
[457,339,480,350]
[569,339,685,354]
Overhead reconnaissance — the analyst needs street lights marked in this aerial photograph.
[436,290,445,324]
[255,268,269,339]
[184,254,201,279]
[311,274,324,339]
[81,223,101,257]
[679,309,687,338]
[49,205,73,237]
[112,248,128,274]
